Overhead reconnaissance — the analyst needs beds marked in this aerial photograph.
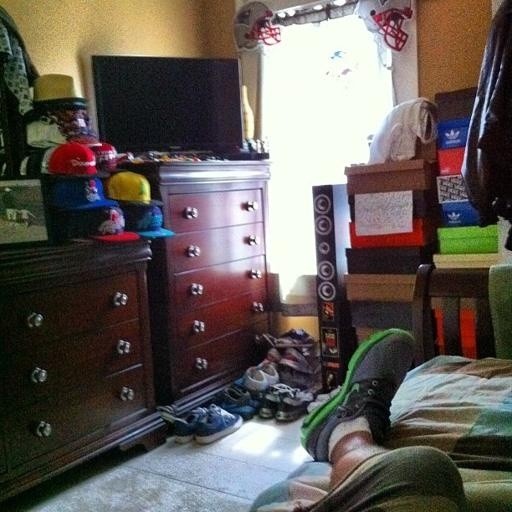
[250,262,510,511]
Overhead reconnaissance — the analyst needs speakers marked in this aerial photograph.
[313,184,352,302]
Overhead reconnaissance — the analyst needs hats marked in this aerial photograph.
[15,74,175,244]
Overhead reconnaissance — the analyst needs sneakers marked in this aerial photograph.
[299,330,416,463]
[174,404,243,445]
[212,329,341,422]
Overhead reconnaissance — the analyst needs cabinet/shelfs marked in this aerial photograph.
[0,240,171,503]
[118,159,270,415]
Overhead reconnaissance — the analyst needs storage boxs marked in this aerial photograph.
[342,85,498,342]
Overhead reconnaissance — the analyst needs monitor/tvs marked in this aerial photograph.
[90,55,244,151]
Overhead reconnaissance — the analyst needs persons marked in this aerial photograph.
[297,325,470,511]
